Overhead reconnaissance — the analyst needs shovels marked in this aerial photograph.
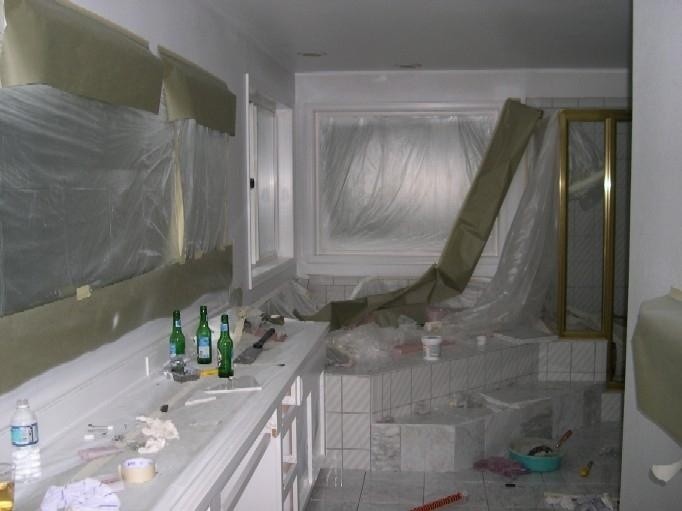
[232,328,275,364]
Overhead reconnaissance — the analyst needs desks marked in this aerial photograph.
[0,304,334,511]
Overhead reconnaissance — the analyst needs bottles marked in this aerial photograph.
[11,399,44,487]
[169,309,186,375]
[196,304,213,363]
[215,314,235,380]
[579,461,594,478]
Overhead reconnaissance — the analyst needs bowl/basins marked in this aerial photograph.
[424,307,448,322]
[505,437,567,472]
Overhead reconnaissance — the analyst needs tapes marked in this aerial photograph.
[122,459,156,483]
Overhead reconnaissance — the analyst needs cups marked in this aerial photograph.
[1,462,17,511]
[476,335,487,346]
